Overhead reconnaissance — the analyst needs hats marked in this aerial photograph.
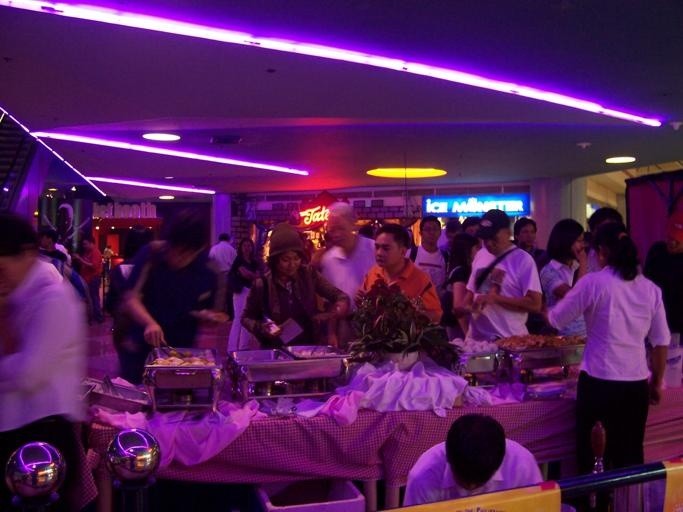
[268,223,306,258]
[475,209,511,239]
[666,210,683,243]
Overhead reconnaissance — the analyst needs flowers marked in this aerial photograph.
[343,273,462,375]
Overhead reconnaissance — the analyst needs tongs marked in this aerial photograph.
[104,374,119,395]
[157,342,186,359]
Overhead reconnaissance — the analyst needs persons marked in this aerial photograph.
[401,414,543,508]
[0,209,88,469]
[32,201,683,388]
[539,219,674,475]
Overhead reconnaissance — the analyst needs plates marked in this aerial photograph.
[190,310,229,323]
[527,381,568,400]
